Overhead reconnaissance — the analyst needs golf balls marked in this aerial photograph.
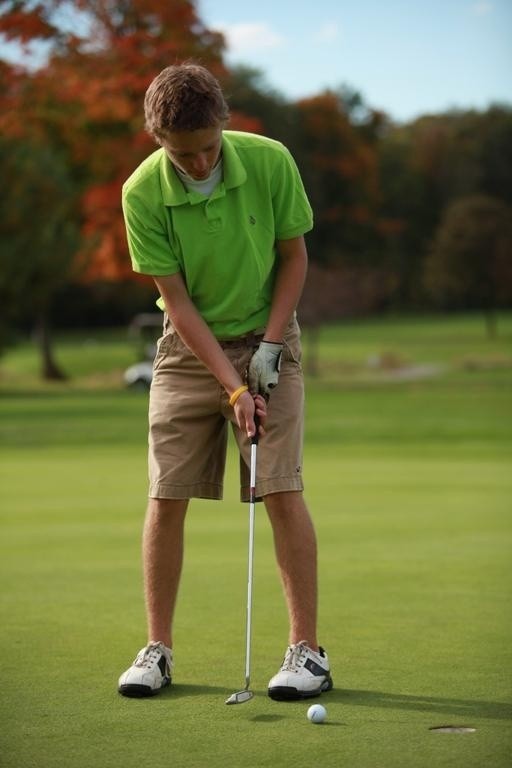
[308,705,326,725]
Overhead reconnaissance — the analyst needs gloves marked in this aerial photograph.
[248,341,282,403]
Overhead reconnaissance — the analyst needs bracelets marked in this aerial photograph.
[228,384,250,408]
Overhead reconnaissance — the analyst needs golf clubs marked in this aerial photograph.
[227,396,258,707]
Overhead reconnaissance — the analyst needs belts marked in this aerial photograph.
[237,312,298,337]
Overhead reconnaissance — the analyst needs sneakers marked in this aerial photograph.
[117,645,174,696]
[267,646,333,700]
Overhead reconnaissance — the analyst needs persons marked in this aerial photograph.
[111,62,335,700]
